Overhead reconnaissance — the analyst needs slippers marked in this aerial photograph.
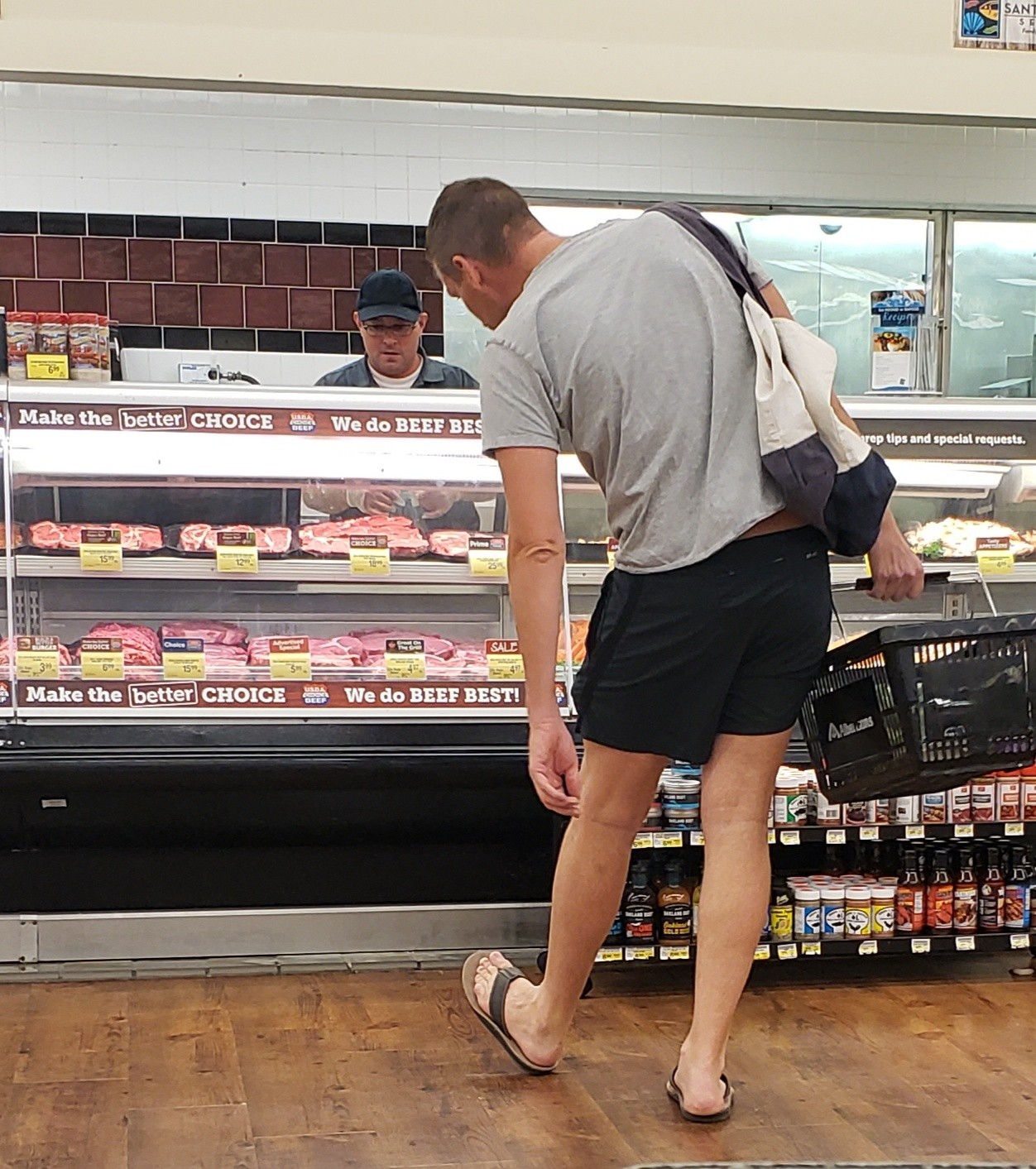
[462,949,559,1075]
[666,1067,735,1122]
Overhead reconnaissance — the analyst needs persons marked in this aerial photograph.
[303,268,481,531]
[426,177,925,1123]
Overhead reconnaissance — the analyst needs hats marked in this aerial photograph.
[355,269,423,322]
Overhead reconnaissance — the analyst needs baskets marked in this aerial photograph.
[798,571,1035,803]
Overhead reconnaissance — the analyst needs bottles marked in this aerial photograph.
[824,840,895,877]
[0,307,122,382]
[896,836,1036,936]
[603,857,702,946]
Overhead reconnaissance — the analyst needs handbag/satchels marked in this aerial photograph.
[645,198,897,558]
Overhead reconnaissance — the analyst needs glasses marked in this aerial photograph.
[362,319,417,338]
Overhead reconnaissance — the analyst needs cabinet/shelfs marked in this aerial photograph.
[533,819,1036,998]
[0,376,1036,965]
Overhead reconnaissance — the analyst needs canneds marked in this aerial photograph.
[768,872,897,945]
[0,305,112,383]
[639,757,700,830]
[768,765,1036,826]
